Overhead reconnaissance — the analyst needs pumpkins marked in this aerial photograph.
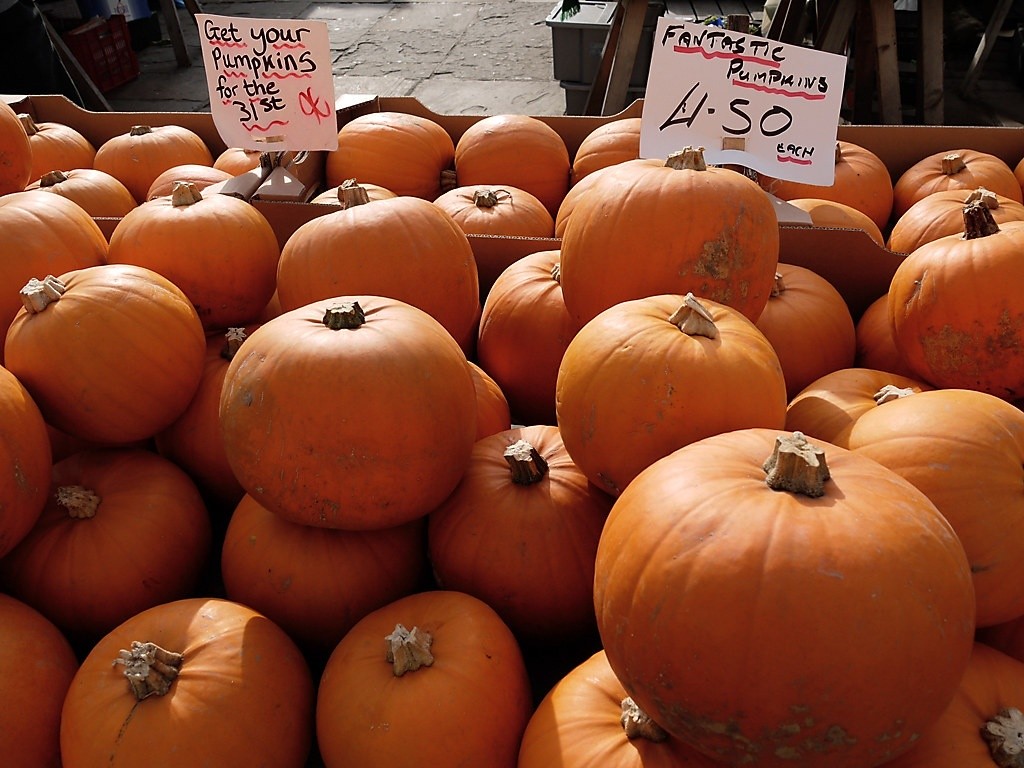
[0,104,1024,768]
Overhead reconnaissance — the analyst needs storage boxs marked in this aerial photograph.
[0,91,282,244]
[247,91,645,303]
[721,124,1024,327]
[4,12,141,98]
[545,0,664,117]
